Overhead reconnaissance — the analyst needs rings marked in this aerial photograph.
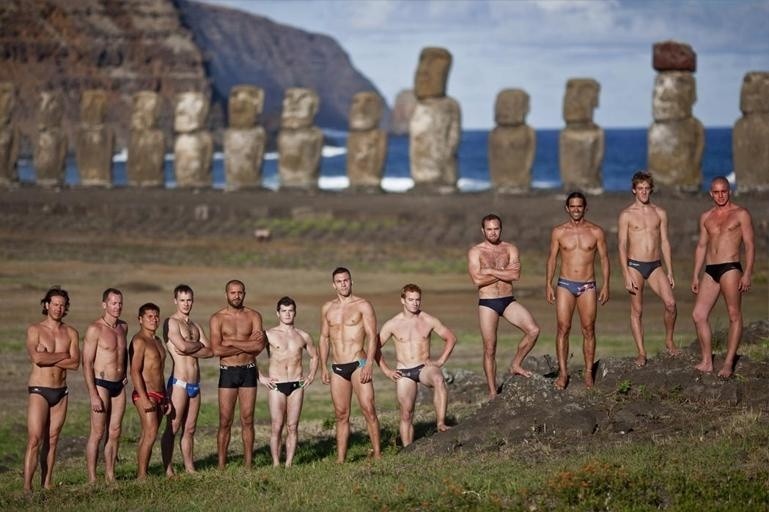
[99,407,102,410]
[364,376,367,378]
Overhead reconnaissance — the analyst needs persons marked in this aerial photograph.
[209,279,266,471]
[619,172,678,367]
[25,285,81,490]
[129,302,172,482]
[546,191,610,389]
[81,288,127,485]
[374,284,457,448]
[253,296,318,468]
[468,213,540,400]
[161,284,215,477]
[318,267,381,464]
[690,176,756,378]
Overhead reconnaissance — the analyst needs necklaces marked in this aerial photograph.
[102,317,118,329]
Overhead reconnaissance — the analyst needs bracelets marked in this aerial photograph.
[501,266,504,270]
